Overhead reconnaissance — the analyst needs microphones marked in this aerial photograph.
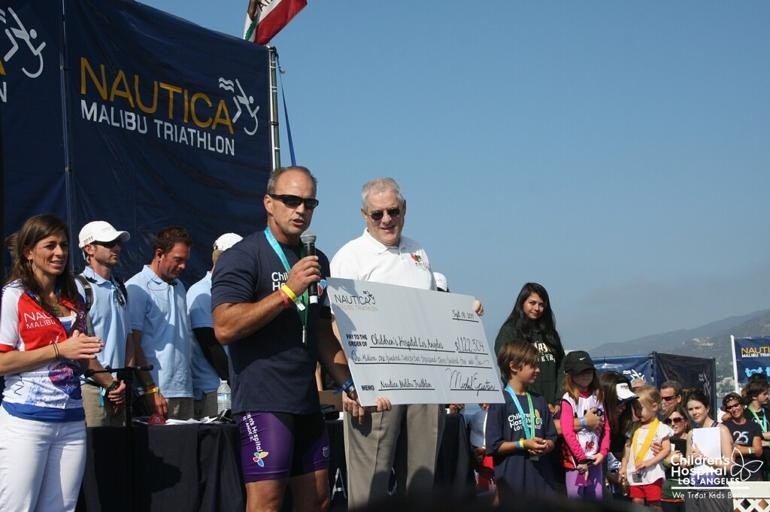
[93,364,153,373]
[300,228,321,304]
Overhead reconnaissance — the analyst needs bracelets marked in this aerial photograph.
[346,385,355,397]
[53,343,60,359]
[143,381,159,397]
[333,378,352,395]
[278,289,293,309]
[107,379,121,394]
[281,285,305,311]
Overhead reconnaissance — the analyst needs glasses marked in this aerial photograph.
[267,191,319,211]
[727,403,741,409]
[94,238,121,248]
[365,206,401,219]
[660,394,678,402]
[664,416,684,425]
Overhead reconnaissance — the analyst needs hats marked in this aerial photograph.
[564,350,596,377]
[213,232,244,252]
[77,220,129,248]
[614,383,639,400]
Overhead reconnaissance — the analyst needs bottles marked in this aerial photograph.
[218,379,231,414]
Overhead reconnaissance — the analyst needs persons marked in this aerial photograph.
[73,221,136,427]
[210,165,392,512]
[185,233,244,419]
[0,213,105,512]
[123,226,194,420]
[434,274,769,512]
[329,176,484,512]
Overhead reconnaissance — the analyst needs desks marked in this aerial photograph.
[74,417,351,512]
